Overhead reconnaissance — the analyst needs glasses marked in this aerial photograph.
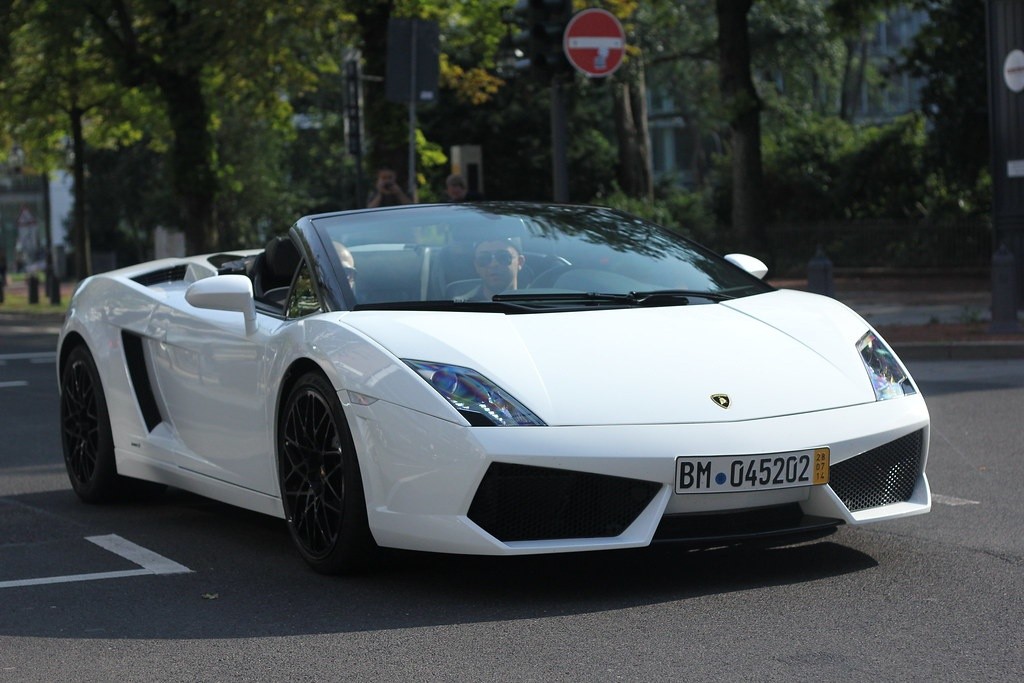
[475,249,518,268]
[344,266,357,282]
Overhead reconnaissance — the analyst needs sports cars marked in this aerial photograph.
[56,199,933,577]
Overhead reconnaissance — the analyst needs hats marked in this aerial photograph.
[471,236,523,256]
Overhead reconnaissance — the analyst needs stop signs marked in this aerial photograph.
[562,8,629,80]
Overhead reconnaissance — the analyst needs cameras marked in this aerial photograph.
[384,181,394,191]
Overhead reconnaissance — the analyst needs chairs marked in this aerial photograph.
[263,234,304,290]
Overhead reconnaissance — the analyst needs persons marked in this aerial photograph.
[15,244,29,272]
[367,165,414,209]
[452,236,525,302]
[446,174,490,201]
[296,239,355,296]
